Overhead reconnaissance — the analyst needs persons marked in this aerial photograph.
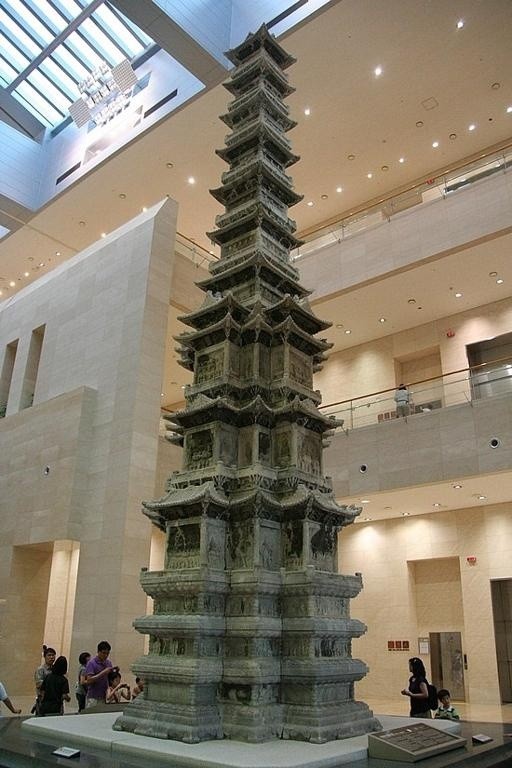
[1,679,22,715]
[30,641,144,716]
[394,382,412,418]
[434,689,459,720]
[400,658,433,719]
[419,402,432,412]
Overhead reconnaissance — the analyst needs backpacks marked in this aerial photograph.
[417,676,439,711]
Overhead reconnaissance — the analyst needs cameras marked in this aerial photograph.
[120,684,127,687]
[112,665,118,672]
